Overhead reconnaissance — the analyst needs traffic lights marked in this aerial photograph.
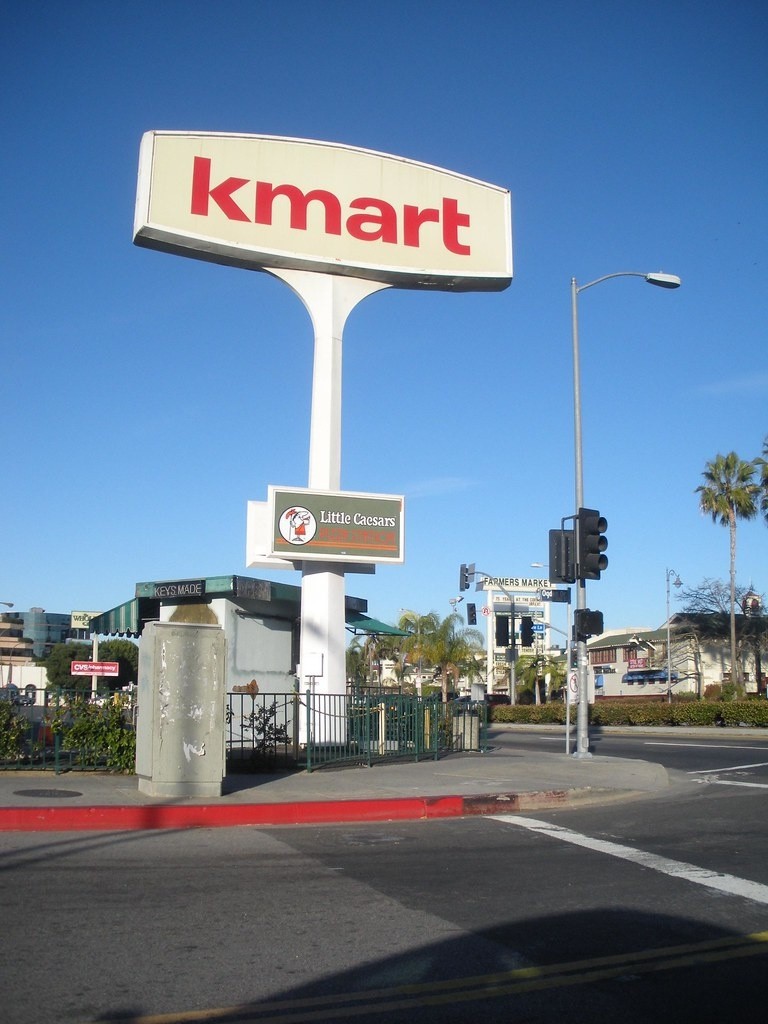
[521,616,535,648]
[467,602,477,625]
[578,506,609,588]
[460,564,470,592]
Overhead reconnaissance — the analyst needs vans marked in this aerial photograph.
[88,691,134,711]
[427,692,511,708]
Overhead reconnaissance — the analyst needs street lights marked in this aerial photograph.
[666,569,685,704]
[570,271,682,757]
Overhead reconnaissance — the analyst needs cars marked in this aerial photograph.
[356,693,417,743]
[20,695,32,706]
